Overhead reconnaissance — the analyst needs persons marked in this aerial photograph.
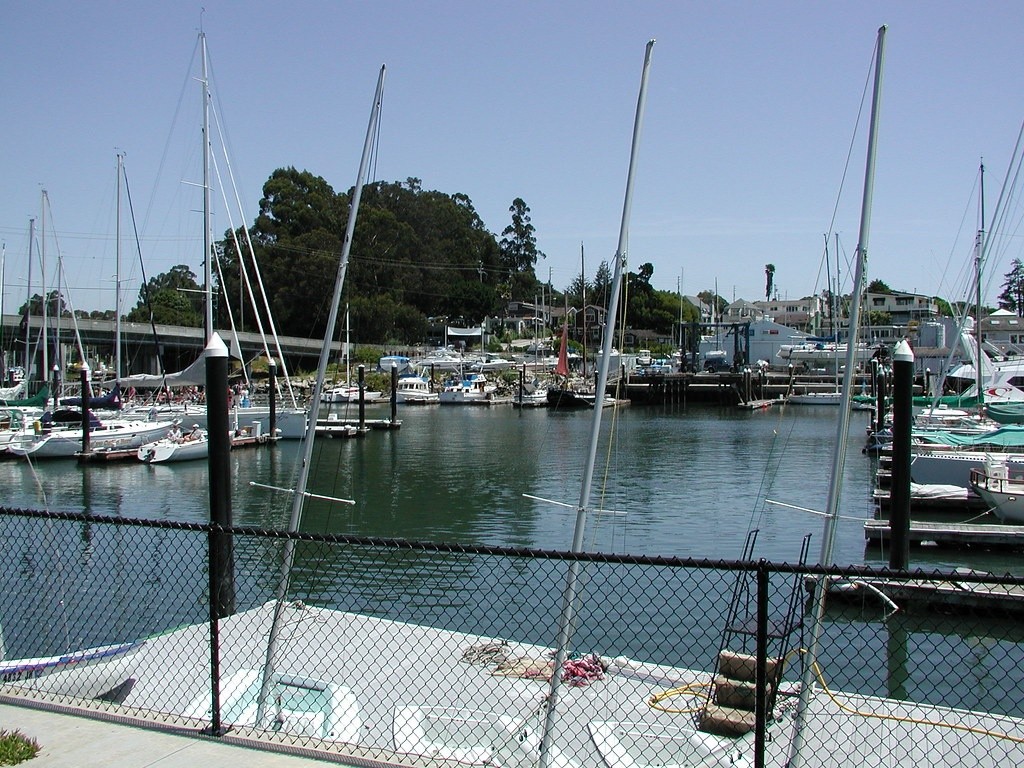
[167,424,202,444]
[129,380,255,408]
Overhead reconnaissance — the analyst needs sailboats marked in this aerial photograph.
[1,0,1024,529]
